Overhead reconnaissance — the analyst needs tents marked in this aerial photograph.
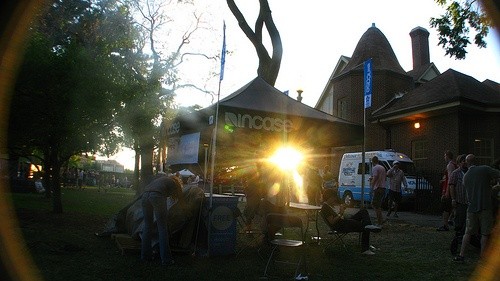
[162,77,361,195]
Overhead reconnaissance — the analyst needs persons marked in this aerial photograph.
[35,150,500,263]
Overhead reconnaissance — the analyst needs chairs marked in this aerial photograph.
[263,213,307,281]
[231,206,264,258]
[319,210,352,255]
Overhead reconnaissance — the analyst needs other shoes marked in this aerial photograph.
[452,255,464,261]
[364,224,381,233]
[448,220,456,226]
[435,226,450,232]
[361,250,376,256]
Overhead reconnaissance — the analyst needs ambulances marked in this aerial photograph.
[338,148,433,211]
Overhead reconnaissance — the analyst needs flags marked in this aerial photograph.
[364,60,372,109]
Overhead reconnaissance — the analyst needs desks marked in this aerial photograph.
[286,202,322,246]
[199,193,237,258]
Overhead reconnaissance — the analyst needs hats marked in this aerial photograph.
[393,160,400,165]
